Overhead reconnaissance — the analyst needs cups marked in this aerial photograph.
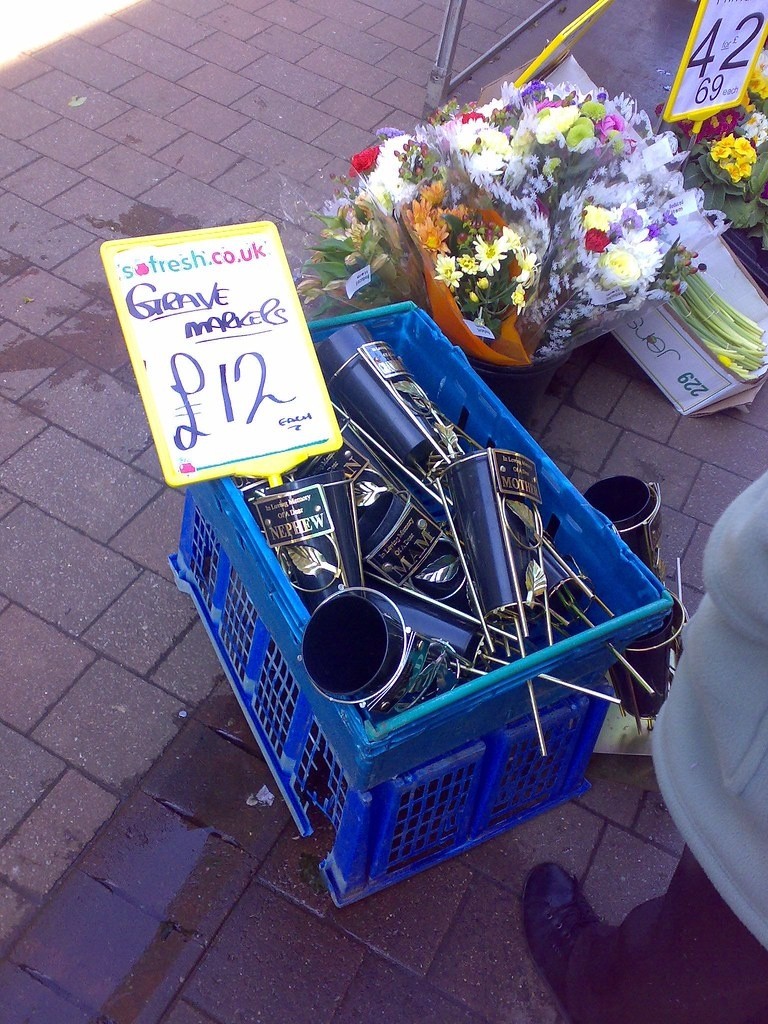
[238,320,683,726]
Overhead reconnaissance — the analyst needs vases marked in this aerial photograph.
[468,349,573,428]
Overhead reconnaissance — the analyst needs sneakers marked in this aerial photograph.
[520,861,606,1023]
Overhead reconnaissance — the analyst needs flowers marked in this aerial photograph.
[278,44,768,344]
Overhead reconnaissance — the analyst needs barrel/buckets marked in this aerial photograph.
[456,342,570,429]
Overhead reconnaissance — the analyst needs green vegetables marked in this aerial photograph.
[660,269,768,381]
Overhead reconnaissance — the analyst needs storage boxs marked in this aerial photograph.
[168,299,680,911]
[481,48,768,417]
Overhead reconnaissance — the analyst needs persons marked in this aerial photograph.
[522,470,768,1024]
